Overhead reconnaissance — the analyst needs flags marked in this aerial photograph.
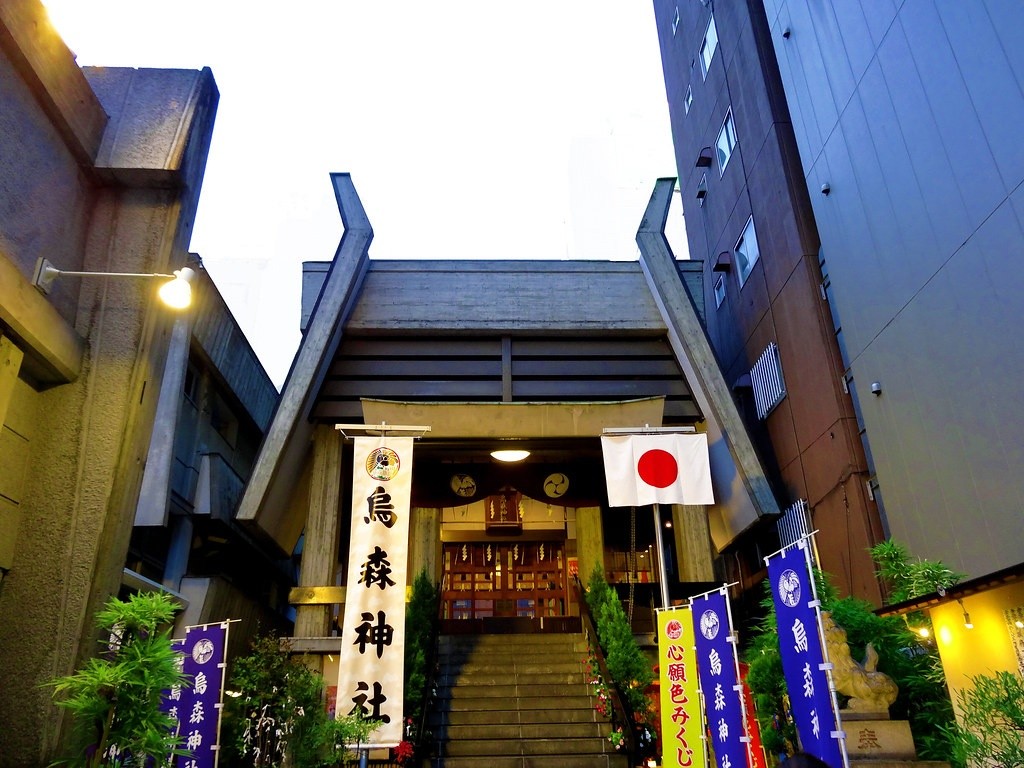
[600,431,716,508]
